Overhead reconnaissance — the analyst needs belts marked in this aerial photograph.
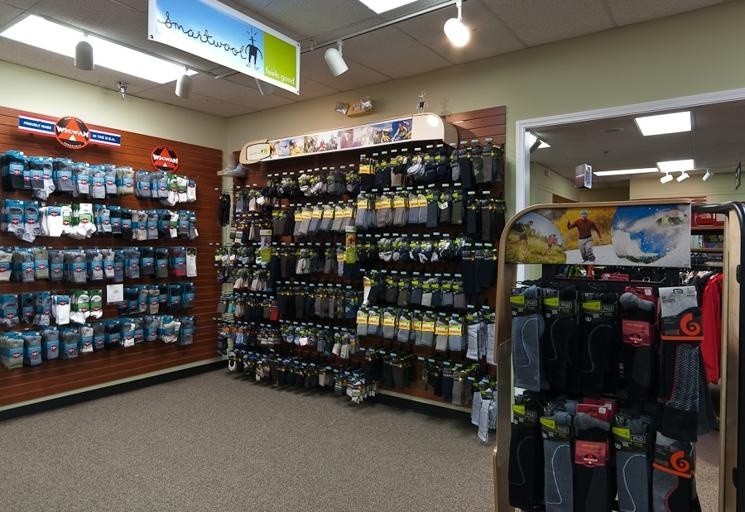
[578,235,592,241]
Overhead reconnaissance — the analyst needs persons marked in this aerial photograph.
[567,209,603,264]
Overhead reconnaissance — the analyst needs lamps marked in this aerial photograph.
[175,65,193,98]
[443,1,471,50]
[74,31,95,72]
[323,40,348,79]
[659,172,674,185]
[676,171,689,183]
[334,95,377,119]
[702,168,710,182]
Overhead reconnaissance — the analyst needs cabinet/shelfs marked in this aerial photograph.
[482,199,740,512]
